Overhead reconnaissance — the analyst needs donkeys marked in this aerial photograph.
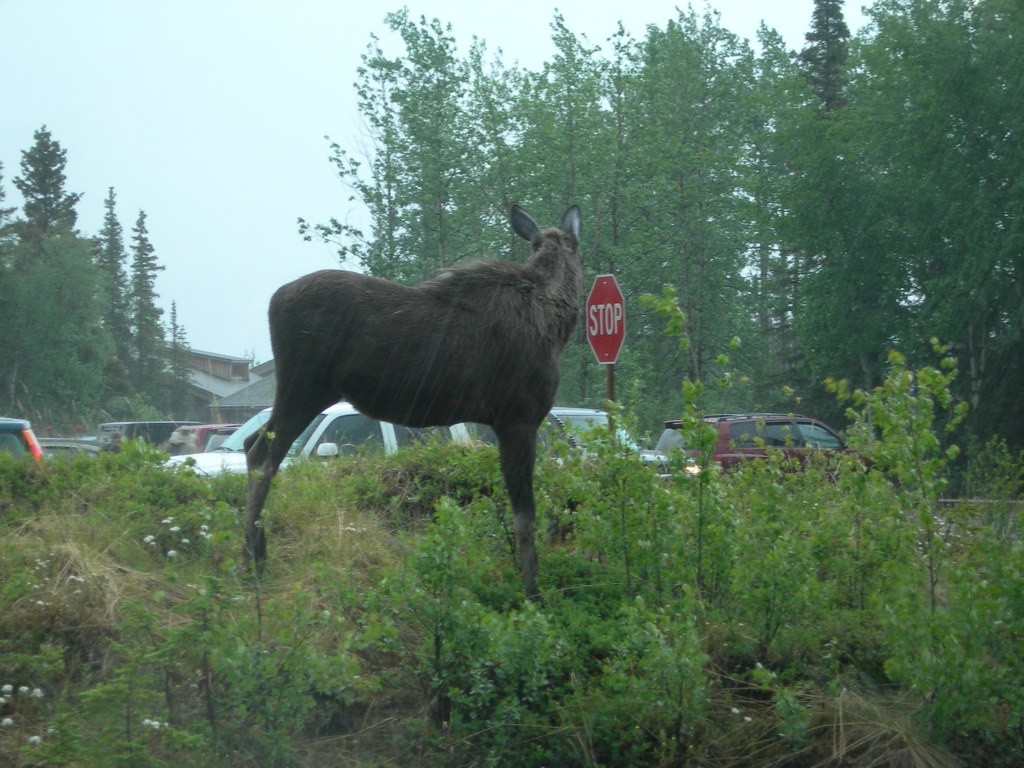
[239,205,584,604]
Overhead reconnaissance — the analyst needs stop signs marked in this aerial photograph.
[585,275,625,366]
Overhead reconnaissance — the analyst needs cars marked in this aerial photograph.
[36,436,104,463]
[484,409,667,483]
[166,423,245,460]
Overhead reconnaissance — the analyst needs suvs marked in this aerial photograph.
[153,401,477,498]
[0,417,47,464]
[205,427,243,457]
[97,420,189,458]
[655,415,902,492]
